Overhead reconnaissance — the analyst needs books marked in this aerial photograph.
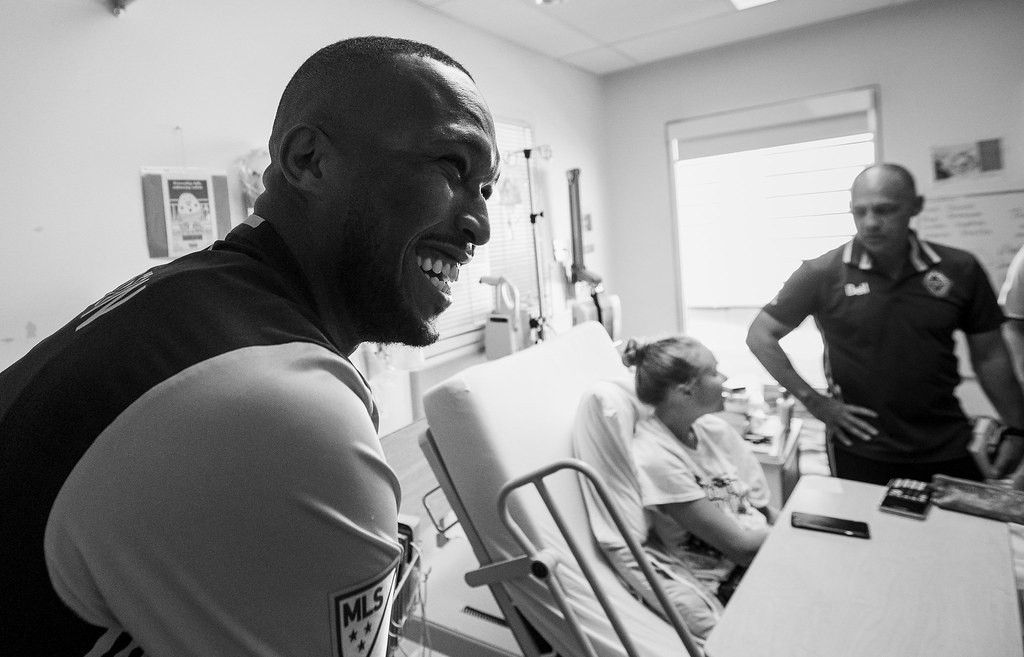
[931,474,1024,524]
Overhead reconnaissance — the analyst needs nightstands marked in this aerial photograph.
[743,415,805,511]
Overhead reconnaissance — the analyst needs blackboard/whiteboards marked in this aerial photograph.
[908,186,1024,381]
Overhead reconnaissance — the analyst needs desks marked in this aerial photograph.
[703,475,1024,656]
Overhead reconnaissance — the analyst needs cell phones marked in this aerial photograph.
[791,512,869,539]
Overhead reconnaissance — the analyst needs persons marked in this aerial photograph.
[998,246,1024,384]
[622,334,781,607]
[746,164,1024,485]
[0,35,502,656]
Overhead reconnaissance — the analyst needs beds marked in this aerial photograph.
[416,318,724,657]
